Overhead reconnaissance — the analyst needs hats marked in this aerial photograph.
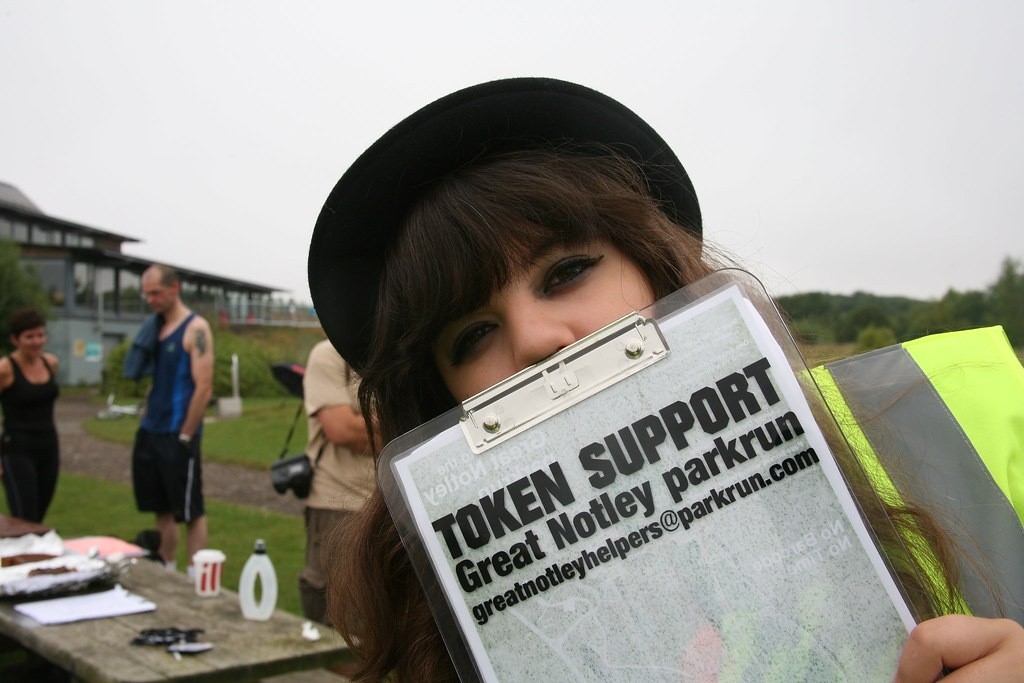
[306,77,703,418]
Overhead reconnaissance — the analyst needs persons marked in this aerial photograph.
[308,76,1023,683]
[293,337,381,631]
[1,308,63,529]
[132,263,216,577]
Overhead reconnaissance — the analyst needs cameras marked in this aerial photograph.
[269,454,315,498]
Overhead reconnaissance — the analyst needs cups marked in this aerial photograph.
[192,549,226,598]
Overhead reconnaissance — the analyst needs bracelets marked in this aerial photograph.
[177,433,193,443]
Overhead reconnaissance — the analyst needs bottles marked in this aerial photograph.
[239,538,280,621]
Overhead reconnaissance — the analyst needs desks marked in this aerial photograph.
[0,512,59,537]
[0,557,360,683]
